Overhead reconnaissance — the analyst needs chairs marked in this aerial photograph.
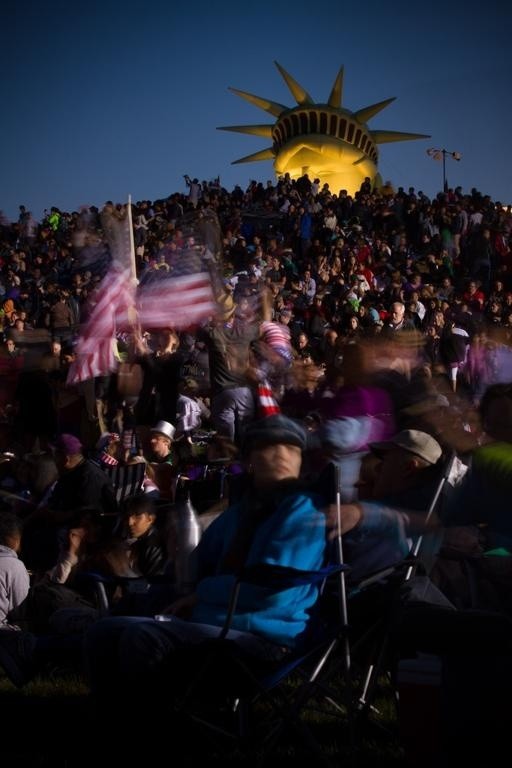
[71,456,355,766]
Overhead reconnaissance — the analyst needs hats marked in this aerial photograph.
[243,414,306,451]
[366,429,443,468]
[150,420,176,442]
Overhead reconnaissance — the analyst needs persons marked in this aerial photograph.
[1,170,512,768]
[274,134,377,197]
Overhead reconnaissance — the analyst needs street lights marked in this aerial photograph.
[426,147,462,192]
